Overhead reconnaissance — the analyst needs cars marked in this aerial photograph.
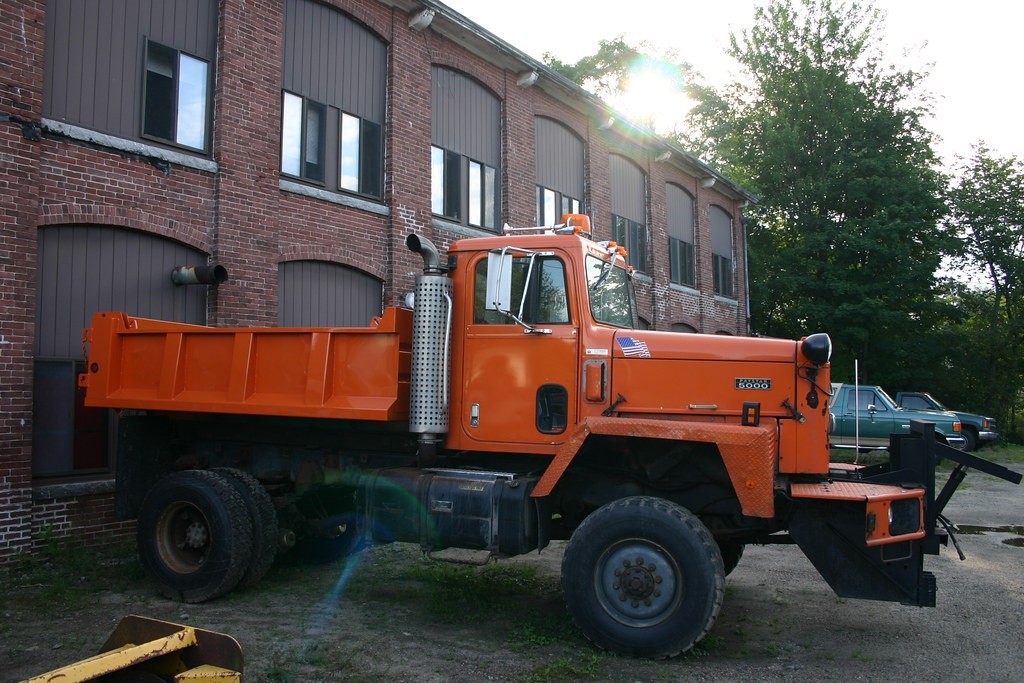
[829,382,968,453]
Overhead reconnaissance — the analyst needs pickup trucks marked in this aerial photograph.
[896,391,999,452]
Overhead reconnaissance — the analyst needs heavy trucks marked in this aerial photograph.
[77,213,1023,663]
